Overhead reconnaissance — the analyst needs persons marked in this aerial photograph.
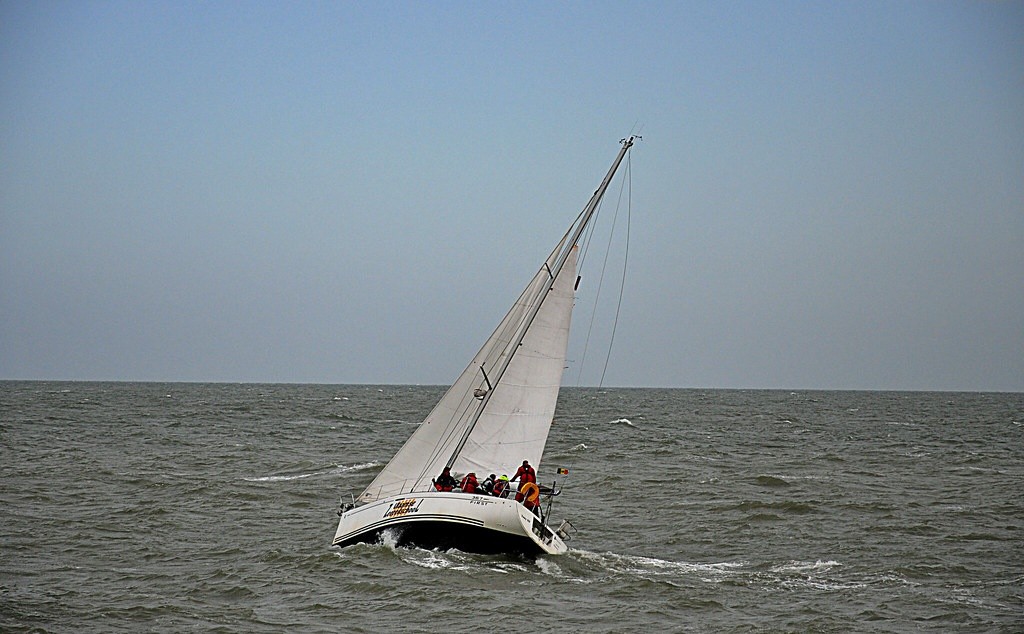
[435,467,457,492]
[480,474,496,495]
[460,473,479,493]
[510,461,536,503]
[493,475,510,498]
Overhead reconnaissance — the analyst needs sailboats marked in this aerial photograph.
[329,130,647,561]
